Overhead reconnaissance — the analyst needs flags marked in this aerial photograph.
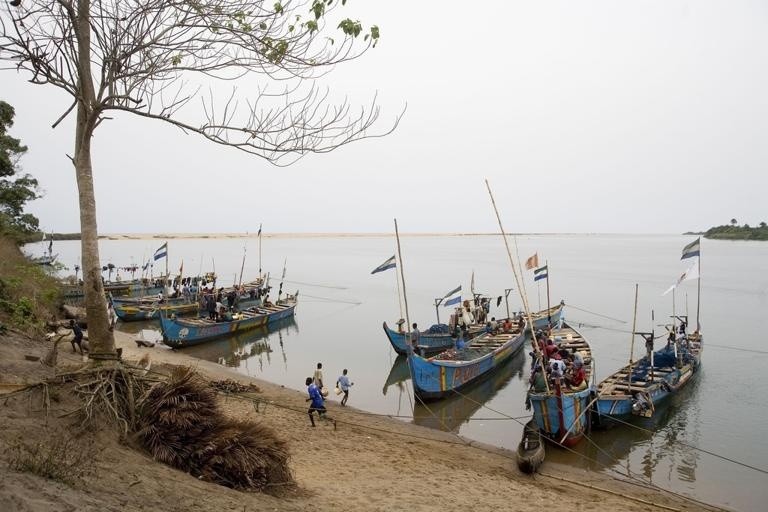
[533,264,548,281]
[443,285,463,307]
[680,236,700,260]
[153,242,167,261]
[524,252,539,271]
[370,253,397,275]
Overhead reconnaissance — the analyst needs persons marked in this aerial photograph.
[151,277,275,323]
[61,319,84,356]
[304,376,337,429]
[335,368,355,408]
[311,361,325,401]
[410,296,589,392]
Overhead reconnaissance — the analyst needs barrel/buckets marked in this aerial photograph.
[567,334,572,340]
[321,387,328,395]
[334,387,341,394]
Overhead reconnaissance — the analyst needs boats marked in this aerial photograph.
[593,236,705,421]
[28,222,302,350]
[485,176,596,449]
[393,217,528,402]
[373,250,565,356]
